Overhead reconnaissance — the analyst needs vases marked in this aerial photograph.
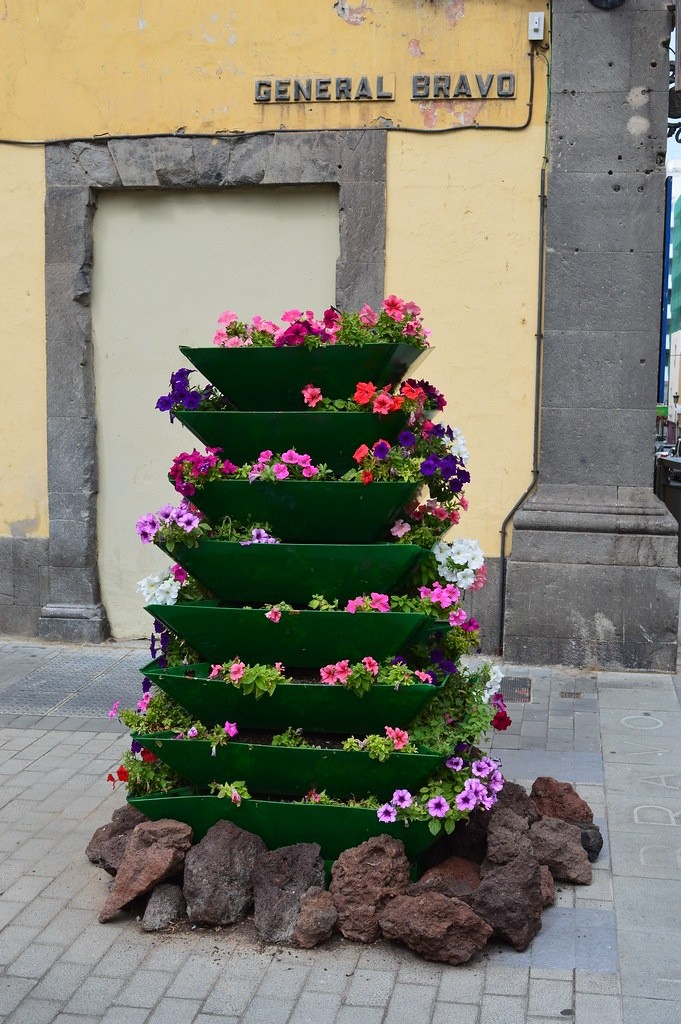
[102,294,514,859]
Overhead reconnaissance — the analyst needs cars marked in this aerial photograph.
[655,436,681,523]
